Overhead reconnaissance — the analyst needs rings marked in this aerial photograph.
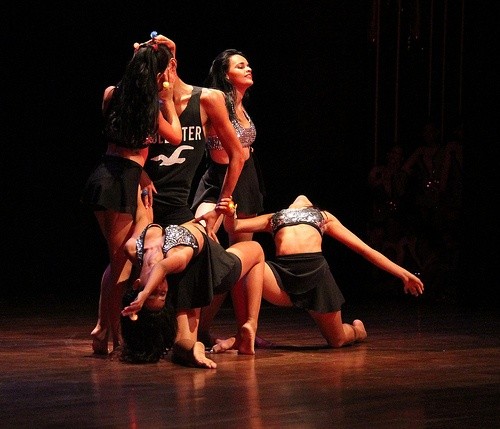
[229,204,235,208]
[142,190,148,195]
[163,82,169,87]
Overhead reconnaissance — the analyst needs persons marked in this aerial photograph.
[362,121,467,310]
[218,195,425,354]
[87,31,274,368]
